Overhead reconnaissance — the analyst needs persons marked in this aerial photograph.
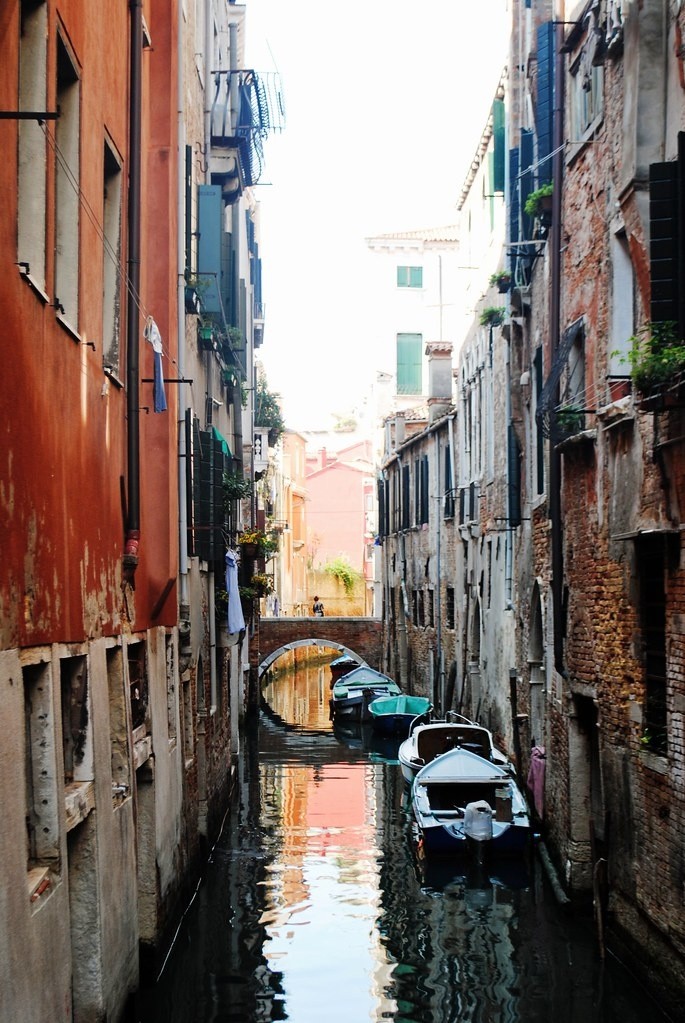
[313,596,324,617]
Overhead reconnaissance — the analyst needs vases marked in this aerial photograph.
[638,376,685,409]
[607,378,629,401]
[246,543,255,556]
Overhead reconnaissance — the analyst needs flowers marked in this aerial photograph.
[215,574,274,603]
[238,524,267,545]
[610,319,685,390]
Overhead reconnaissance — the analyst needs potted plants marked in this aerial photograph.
[556,404,585,442]
[197,313,248,407]
[479,306,507,327]
[488,270,513,293]
[522,177,554,216]
[184,274,210,314]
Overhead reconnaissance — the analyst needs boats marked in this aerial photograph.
[408,745,531,866]
[332,665,401,721]
[367,692,434,737]
[330,653,360,685]
[398,711,517,788]
